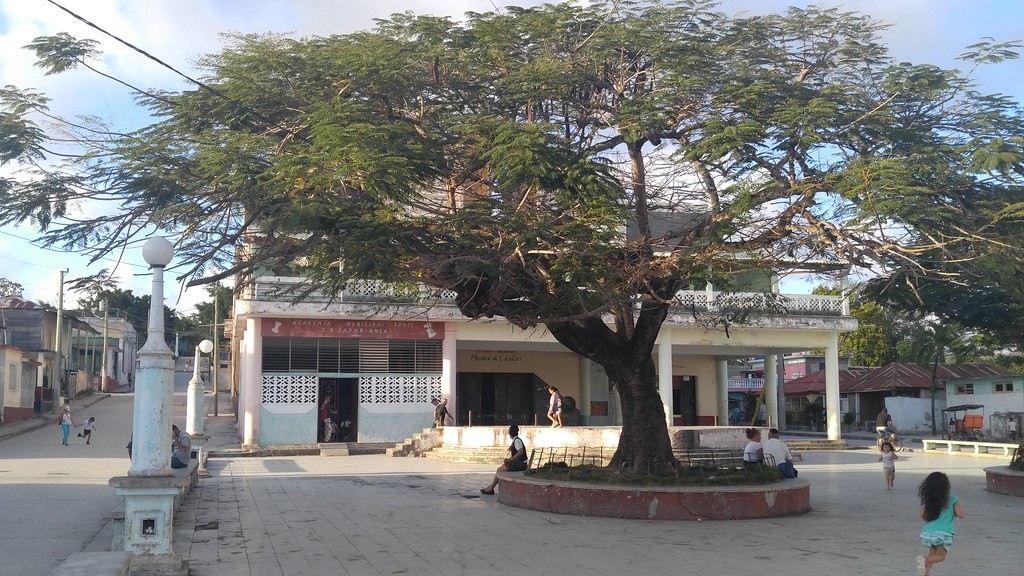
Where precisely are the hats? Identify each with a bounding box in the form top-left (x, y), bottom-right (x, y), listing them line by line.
top-left (430, 398), bottom-right (437, 403)
top-left (882, 408), bottom-right (887, 413)
top-left (64, 406), bottom-right (70, 411)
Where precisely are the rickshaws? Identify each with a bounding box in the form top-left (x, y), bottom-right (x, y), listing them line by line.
top-left (940, 401), bottom-right (983, 442)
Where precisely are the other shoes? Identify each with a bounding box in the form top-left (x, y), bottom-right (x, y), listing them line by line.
top-left (480, 489), bottom-right (494, 495)
top-left (78, 433), bottom-right (81, 437)
top-left (66, 443), bottom-right (68, 446)
top-left (917, 555), bottom-right (928, 576)
top-left (886, 485), bottom-right (890, 489)
top-left (63, 438), bottom-right (65, 444)
top-left (890, 481), bottom-right (894, 487)
top-left (554, 422), bottom-right (560, 428)
top-left (86, 442), bottom-right (90, 444)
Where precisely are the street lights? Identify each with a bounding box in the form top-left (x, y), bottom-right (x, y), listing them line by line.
top-left (186, 340), bottom-right (214, 436)
top-left (125, 235), bottom-right (177, 478)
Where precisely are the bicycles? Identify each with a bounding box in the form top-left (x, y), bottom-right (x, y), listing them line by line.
top-left (874, 427), bottom-right (903, 455)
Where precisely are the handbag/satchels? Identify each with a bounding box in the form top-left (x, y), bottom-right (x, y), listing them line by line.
top-left (432, 421), bottom-right (437, 427)
top-left (553, 404), bottom-right (558, 412)
top-left (58, 412), bottom-right (63, 425)
top-left (778, 463), bottom-right (796, 479)
top-left (737, 417), bottom-right (740, 422)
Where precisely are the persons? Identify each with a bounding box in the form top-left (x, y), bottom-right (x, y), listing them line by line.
top-left (172, 424), bottom-right (191, 469)
top-left (879, 442), bottom-right (898, 490)
top-left (430, 398), bottom-right (454, 428)
top-left (876, 409), bottom-right (897, 447)
top-left (743, 428), bottom-right (792, 469)
top-left (61, 406), bottom-right (96, 445)
top-left (126, 434), bottom-right (132, 460)
top-left (1008, 417), bottom-right (1018, 443)
top-left (320, 395), bottom-right (339, 443)
top-left (916, 472), bottom-right (964, 576)
top-left (548, 386), bottom-right (562, 429)
top-left (480, 425), bottom-right (528, 495)
